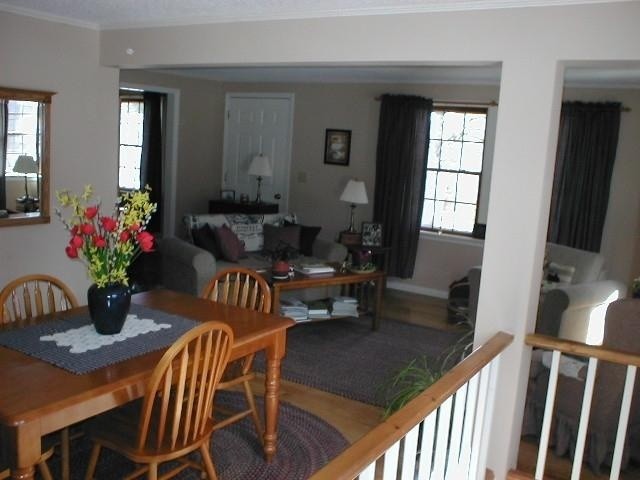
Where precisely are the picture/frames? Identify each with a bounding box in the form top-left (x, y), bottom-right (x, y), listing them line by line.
top-left (323, 126), bottom-right (353, 166)
top-left (219, 188), bottom-right (236, 202)
top-left (361, 220), bottom-right (385, 247)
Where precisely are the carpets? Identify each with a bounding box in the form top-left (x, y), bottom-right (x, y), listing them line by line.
top-left (239, 313), bottom-right (540, 438)
top-left (3, 383), bottom-right (351, 480)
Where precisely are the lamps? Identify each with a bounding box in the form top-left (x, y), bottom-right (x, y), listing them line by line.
top-left (248, 154), bottom-right (274, 205)
top-left (10, 154), bottom-right (38, 216)
top-left (338, 177), bottom-right (369, 236)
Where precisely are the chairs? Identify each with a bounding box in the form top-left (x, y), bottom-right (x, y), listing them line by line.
top-left (1, 271), bottom-right (105, 478)
top-left (525, 298), bottom-right (640, 475)
top-left (0, 442), bottom-right (56, 479)
top-left (78, 320), bottom-right (237, 480)
top-left (166, 271), bottom-right (276, 450)
top-left (464, 239), bottom-right (630, 349)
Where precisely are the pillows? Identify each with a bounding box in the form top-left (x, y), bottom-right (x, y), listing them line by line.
top-left (264, 221), bottom-right (301, 254)
top-left (192, 221), bottom-right (222, 259)
top-left (213, 224), bottom-right (247, 263)
top-left (547, 259), bottom-right (577, 285)
top-left (281, 217), bottom-right (321, 256)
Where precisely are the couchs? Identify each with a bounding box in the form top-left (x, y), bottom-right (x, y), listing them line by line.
top-left (160, 210), bottom-right (353, 310)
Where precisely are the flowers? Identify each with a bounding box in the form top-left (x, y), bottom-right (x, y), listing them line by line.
top-left (53, 178), bottom-right (161, 289)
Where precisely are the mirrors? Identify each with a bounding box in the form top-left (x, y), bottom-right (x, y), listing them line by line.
top-left (0, 86), bottom-right (59, 229)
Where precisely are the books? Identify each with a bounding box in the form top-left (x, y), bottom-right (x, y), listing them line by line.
top-left (279, 296), bottom-right (359, 322)
top-left (296, 261), bottom-right (335, 274)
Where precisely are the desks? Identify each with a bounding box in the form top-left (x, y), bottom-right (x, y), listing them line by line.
top-left (333, 238), bottom-right (394, 305)
top-left (206, 199), bottom-right (280, 216)
top-left (257, 262), bottom-right (391, 334)
top-left (1, 287), bottom-right (296, 478)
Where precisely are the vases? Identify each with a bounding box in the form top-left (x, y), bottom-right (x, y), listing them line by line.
top-left (88, 282), bottom-right (130, 336)
